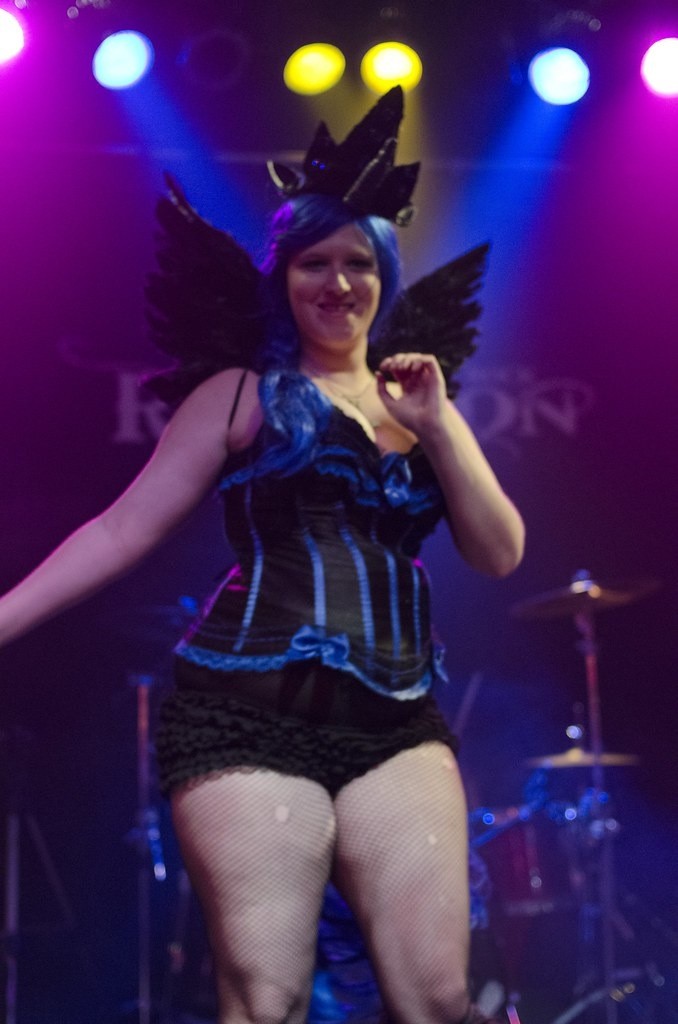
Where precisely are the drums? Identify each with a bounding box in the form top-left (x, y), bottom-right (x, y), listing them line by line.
top-left (468, 804), bottom-right (577, 917)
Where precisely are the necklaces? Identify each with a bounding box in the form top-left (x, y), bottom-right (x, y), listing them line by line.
top-left (304, 364), bottom-right (375, 403)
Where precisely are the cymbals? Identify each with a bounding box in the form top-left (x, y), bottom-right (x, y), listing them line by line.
top-left (511, 573), bottom-right (660, 616)
top-left (516, 747), bottom-right (641, 768)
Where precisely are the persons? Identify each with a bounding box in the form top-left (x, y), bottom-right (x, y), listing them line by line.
top-left (0, 195), bottom-right (526, 1024)
